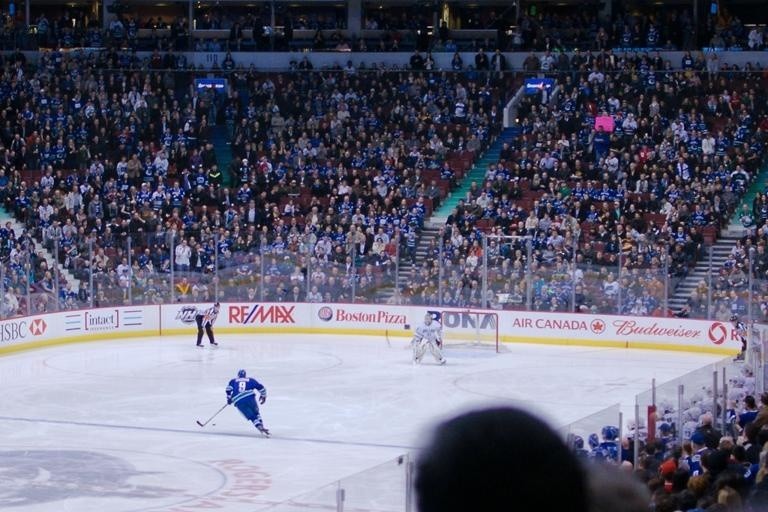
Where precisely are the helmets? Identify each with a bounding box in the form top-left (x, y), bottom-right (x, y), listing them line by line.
top-left (731, 365), bottom-right (755, 401)
top-left (214, 301), bottom-right (220, 311)
top-left (425, 313), bottom-right (431, 325)
top-left (626, 385), bottom-right (722, 439)
top-left (574, 426), bottom-right (619, 448)
top-left (238, 370), bottom-right (246, 377)
top-left (731, 315), bottom-right (737, 321)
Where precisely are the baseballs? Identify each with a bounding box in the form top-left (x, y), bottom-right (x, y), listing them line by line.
top-left (376, 261), bottom-right (379, 265)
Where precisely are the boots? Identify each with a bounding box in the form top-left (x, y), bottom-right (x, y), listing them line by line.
top-left (258, 420), bottom-right (268, 432)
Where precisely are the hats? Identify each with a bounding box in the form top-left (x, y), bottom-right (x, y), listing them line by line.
top-left (691, 433), bottom-right (705, 444)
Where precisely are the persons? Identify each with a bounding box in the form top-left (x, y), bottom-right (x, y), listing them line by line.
top-left (410, 314), bottom-right (446, 365)
top-left (225, 370), bottom-right (270, 435)
top-left (730, 315), bottom-right (762, 362)
top-left (564, 357), bottom-right (768, 512)
top-left (413, 406), bottom-right (591, 512)
top-left (0, 0), bottom-right (768, 322)
top-left (195, 302), bottom-right (222, 347)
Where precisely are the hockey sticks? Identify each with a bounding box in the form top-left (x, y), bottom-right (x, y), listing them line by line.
top-left (196, 402), bottom-right (229, 427)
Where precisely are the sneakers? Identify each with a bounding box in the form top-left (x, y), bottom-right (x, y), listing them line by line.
top-left (733, 354), bottom-right (744, 361)
top-left (197, 342), bottom-right (218, 347)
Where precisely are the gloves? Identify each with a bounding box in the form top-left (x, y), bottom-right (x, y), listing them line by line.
top-left (259, 396), bottom-right (265, 404)
top-left (228, 399), bottom-right (232, 404)
